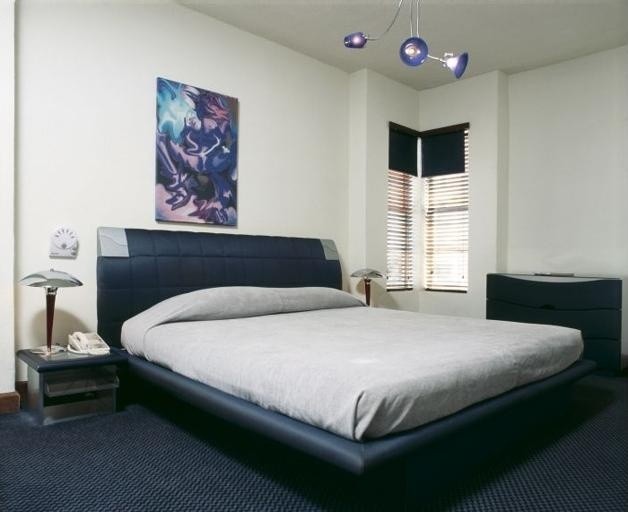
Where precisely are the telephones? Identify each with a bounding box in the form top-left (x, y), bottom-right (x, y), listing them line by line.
top-left (68, 331), bottom-right (111, 355)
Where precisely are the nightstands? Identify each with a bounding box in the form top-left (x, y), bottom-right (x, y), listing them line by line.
top-left (20, 350), bottom-right (126, 422)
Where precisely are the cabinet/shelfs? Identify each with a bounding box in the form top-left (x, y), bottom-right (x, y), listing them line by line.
top-left (486, 273), bottom-right (621, 376)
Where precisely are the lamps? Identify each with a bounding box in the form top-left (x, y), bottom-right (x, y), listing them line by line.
top-left (349, 268), bottom-right (383, 307)
top-left (341, 0), bottom-right (469, 79)
top-left (18, 267), bottom-right (83, 356)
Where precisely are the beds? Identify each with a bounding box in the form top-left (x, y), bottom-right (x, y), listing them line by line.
top-left (96, 228), bottom-right (598, 512)
